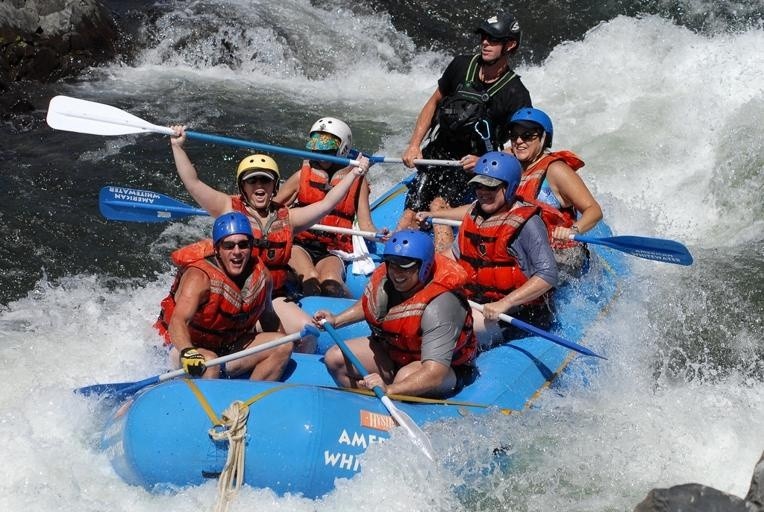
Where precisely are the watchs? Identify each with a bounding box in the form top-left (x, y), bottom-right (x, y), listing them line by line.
top-left (570, 225), bottom-right (581, 233)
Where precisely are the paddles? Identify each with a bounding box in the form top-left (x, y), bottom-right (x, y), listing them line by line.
top-left (345, 149), bottom-right (467, 173)
top-left (466, 296), bottom-right (605, 359)
top-left (74, 325), bottom-right (319, 404)
top-left (45, 96), bottom-right (364, 171)
top-left (416, 214), bottom-right (693, 267)
top-left (99, 185), bottom-right (390, 243)
top-left (317, 315), bottom-right (433, 464)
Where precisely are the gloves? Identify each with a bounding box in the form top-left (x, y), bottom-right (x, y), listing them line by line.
top-left (180, 347), bottom-right (206, 379)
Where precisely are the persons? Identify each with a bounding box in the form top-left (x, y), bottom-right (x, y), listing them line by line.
top-left (169, 125), bottom-right (370, 355)
top-left (151, 212), bottom-right (303, 382)
top-left (270, 117), bottom-right (394, 299)
top-left (441, 151), bottom-right (560, 350)
top-left (394, 10), bottom-right (534, 234)
top-left (415, 108), bottom-right (604, 288)
top-left (311, 229), bottom-right (479, 404)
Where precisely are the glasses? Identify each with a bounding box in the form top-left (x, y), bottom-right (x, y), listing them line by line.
top-left (220, 240), bottom-right (249, 250)
top-left (509, 131), bottom-right (545, 140)
top-left (246, 175), bottom-right (271, 185)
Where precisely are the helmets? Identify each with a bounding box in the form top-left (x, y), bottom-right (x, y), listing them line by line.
top-left (480, 11), bottom-right (520, 44)
top-left (212, 213), bottom-right (254, 246)
top-left (467, 152), bottom-right (522, 203)
top-left (382, 229), bottom-right (434, 283)
top-left (308, 117), bottom-right (352, 158)
top-left (506, 106), bottom-right (553, 146)
top-left (236, 154), bottom-right (280, 185)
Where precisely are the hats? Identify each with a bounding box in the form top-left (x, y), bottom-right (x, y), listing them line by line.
top-left (379, 255), bottom-right (419, 268)
top-left (305, 131), bottom-right (341, 151)
top-left (504, 120), bottom-right (540, 130)
top-left (467, 173), bottom-right (506, 187)
top-left (241, 169), bottom-right (276, 181)
top-left (474, 23), bottom-right (502, 39)
